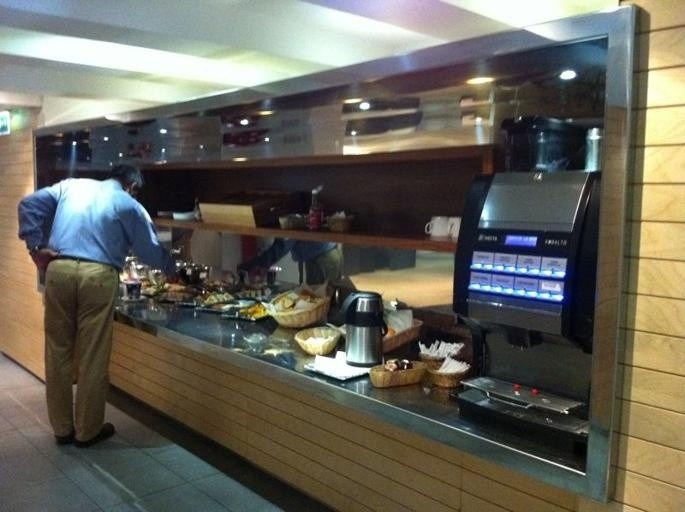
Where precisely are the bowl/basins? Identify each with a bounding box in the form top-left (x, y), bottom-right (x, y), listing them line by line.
top-left (157, 211), bottom-right (196, 222)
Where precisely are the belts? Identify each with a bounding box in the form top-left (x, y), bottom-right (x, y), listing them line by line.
top-left (52, 255), bottom-right (90, 261)
top-left (322, 245), bottom-right (338, 256)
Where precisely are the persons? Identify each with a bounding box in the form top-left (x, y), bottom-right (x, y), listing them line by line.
top-left (236, 237), bottom-right (359, 297)
top-left (16, 164), bottom-right (179, 449)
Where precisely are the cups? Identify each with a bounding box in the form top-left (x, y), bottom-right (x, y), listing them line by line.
top-left (425, 216), bottom-right (462, 237)
top-left (125, 281), bottom-right (142, 298)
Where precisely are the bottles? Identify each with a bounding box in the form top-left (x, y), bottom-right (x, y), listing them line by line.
top-left (308, 188), bottom-right (323, 230)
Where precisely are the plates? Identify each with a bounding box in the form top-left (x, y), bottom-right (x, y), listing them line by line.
top-left (120, 295), bottom-right (147, 302)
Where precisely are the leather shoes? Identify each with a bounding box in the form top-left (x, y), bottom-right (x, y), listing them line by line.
top-left (54, 423), bottom-right (115, 448)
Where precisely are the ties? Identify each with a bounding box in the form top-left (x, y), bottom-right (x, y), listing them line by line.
top-left (298, 258), bottom-right (303, 285)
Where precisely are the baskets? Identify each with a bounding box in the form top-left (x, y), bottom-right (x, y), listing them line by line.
top-left (325, 215), bottom-right (356, 233)
top-left (295, 327), bottom-right (341, 356)
top-left (268, 287), bottom-right (330, 329)
top-left (369, 319), bottom-right (471, 388)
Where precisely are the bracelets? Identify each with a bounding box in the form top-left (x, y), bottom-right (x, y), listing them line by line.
top-left (28, 244), bottom-right (47, 253)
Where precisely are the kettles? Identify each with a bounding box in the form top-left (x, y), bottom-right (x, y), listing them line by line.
top-left (337, 291), bottom-right (389, 366)
